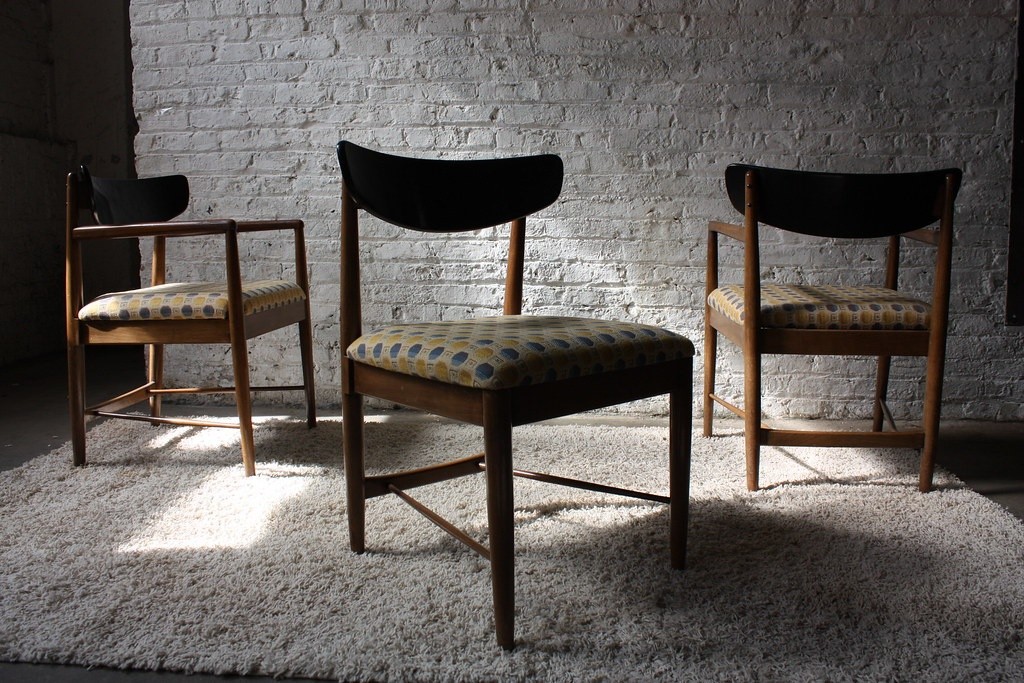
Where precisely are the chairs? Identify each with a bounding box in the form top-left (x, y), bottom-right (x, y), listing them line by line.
top-left (704, 161), bottom-right (965, 493)
top-left (336, 140), bottom-right (695, 651)
top-left (66, 162), bottom-right (317, 474)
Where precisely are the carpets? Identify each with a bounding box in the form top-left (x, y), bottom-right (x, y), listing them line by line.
top-left (0, 412), bottom-right (1024, 682)
